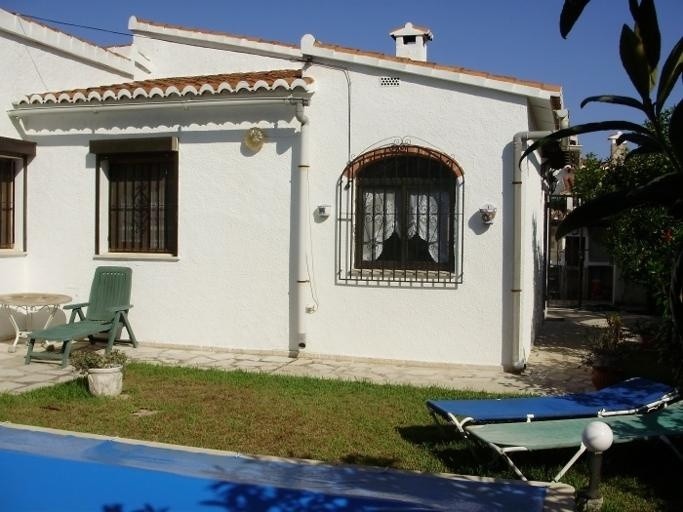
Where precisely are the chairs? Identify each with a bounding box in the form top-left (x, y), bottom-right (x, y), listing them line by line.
top-left (23, 265), bottom-right (137, 369)
top-left (424, 376), bottom-right (683, 482)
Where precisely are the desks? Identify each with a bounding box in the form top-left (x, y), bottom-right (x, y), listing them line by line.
top-left (0, 293), bottom-right (72, 352)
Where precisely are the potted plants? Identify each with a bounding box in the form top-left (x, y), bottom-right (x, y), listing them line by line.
top-left (69, 348), bottom-right (129, 398)
top-left (585, 325), bottom-right (625, 390)
